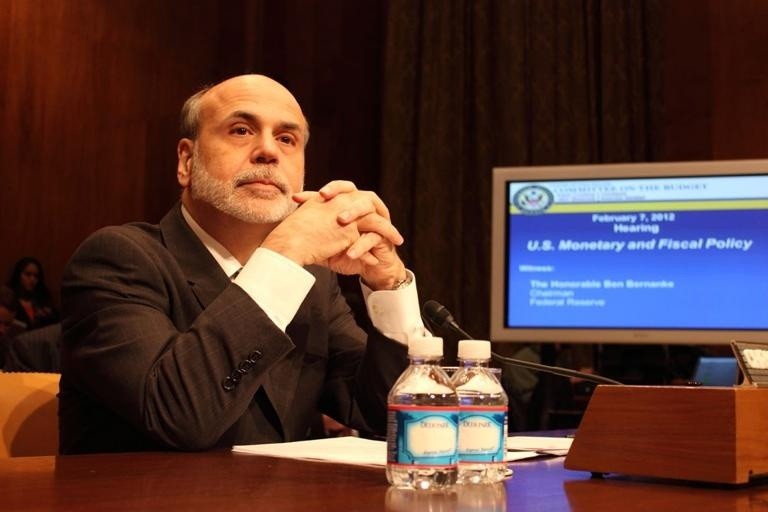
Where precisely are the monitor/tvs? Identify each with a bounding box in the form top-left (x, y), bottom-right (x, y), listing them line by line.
top-left (488, 157), bottom-right (768, 350)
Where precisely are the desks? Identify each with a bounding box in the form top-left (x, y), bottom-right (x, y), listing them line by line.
top-left (0, 429), bottom-right (768, 512)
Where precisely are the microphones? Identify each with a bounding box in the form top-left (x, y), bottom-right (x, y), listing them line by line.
top-left (421, 299), bottom-right (704, 386)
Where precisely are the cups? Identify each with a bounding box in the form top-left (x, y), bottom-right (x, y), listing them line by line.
top-left (435, 364), bottom-right (503, 383)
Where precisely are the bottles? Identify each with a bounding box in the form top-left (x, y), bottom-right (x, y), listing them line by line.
top-left (383, 336), bottom-right (459, 494)
top-left (449, 339), bottom-right (509, 486)
top-left (386, 481), bottom-right (506, 512)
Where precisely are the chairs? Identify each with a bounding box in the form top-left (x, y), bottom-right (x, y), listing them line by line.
top-left (0, 372), bottom-right (359, 460)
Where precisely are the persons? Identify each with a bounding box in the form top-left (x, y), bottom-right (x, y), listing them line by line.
top-left (57, 71), bottom-right (436, 453)
top-left (6, 256), bottom-right (60, 331)
top-left (499, 342), bottom-right (742, 433)
top-left (0, 300), bottom-right (62, 373)
